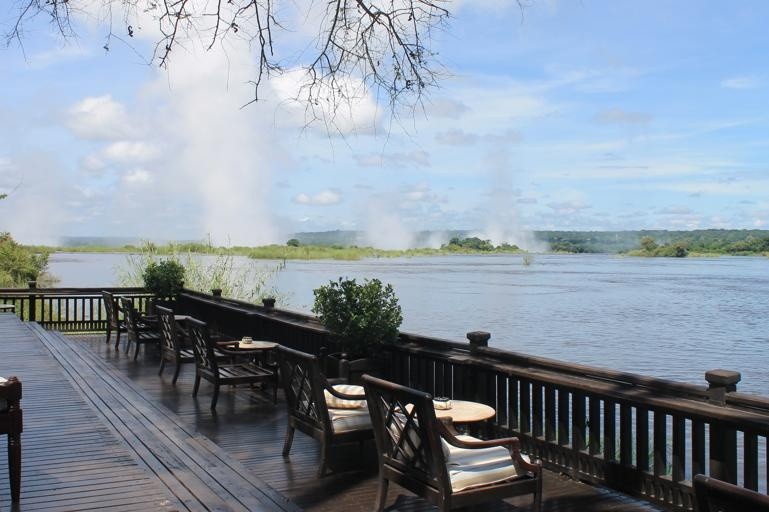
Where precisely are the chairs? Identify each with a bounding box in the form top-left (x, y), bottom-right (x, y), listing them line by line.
top-left (358, 370), bottom-right (543, 510)
top-left (185, 316), bottom-right (275, 413)
top-left (272, 340), bottom-right (379, 480)
top-left (119, 295), bottom-right (162, 361)
top-left (101, 289), bottom-right (146, 349)
top-left (155, 305), bottom-right (234, 387)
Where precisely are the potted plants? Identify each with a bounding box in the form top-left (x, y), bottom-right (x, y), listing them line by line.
top-left (310, 274), bottom-right (403, 383)
top-left (141, 257), bottom-right (189, 315)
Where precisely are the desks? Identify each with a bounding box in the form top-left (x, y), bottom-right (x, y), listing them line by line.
top-left (166, 312), bottom-right (191, 323)
top-left (393, 392), bottom-right (498, 428)
top-left (223, 339), bottom-right (276, 355)
